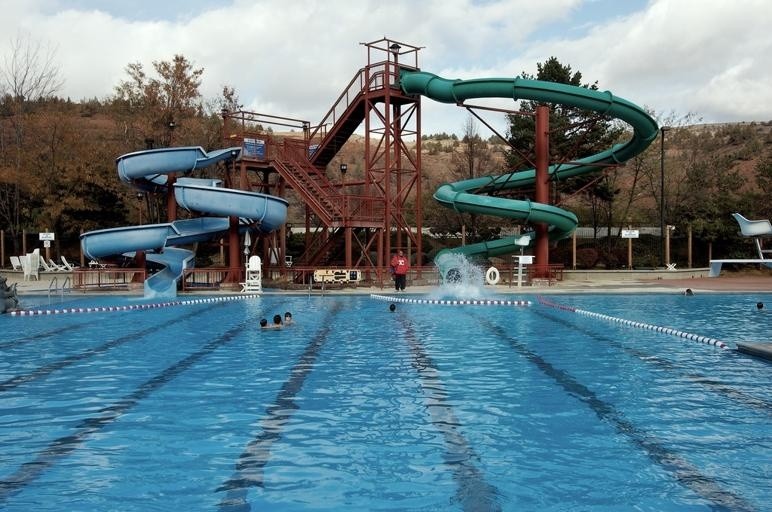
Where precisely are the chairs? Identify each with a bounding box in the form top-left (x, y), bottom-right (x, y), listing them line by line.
top-left (9, 249), bottom-right (80, 281)
top-left (240, 256), bottom-right (262, 293)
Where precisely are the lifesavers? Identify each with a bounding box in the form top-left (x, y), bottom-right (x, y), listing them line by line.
top-left (485, 266), bottom-right (500, 285)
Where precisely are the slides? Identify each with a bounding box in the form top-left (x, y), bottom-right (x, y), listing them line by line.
top-left (80, 146), bottom-right (289, 299)
top-left (399, 71), bottom-right (659, 286)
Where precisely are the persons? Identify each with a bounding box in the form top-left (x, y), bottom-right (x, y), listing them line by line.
top-left (271, 315), bottom-right (285, 327)
top-left (282, 311), bottom-right (296, 325)
top-left (757, 302), bottom-right (763, 309)
top-left (390, 249), bottom-right (409, 292)
top-left (260, 319), bottom-right (272, 328)
top-left (386, 304), bottom-right (397, 313)
top-left (682, 287), bottom-right (695, 297)
